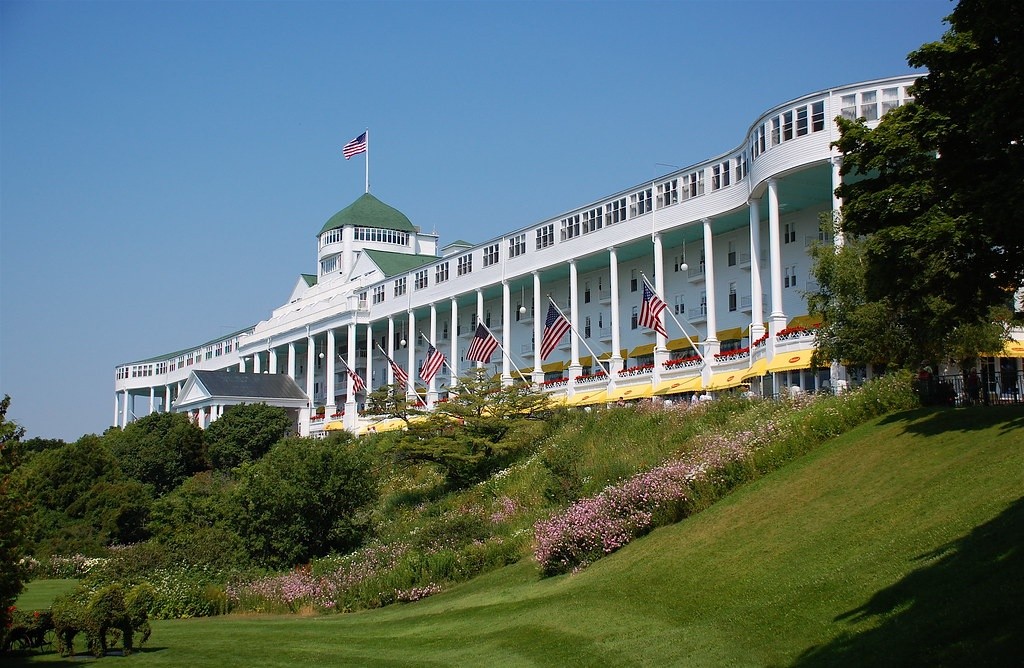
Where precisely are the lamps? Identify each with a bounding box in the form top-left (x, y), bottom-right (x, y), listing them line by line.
top-left (263, 359), bottom-right (268, 374)
top-left (400, 322), bottom-right (406, 345)
top-left (519, 285), bottom-right (526, 314)
top-left (680, 232), bottom-right (689, 270)
top-left (171, 387), bottom-right (176, 404)
top-left (319, 340), bottom-right (324, 358)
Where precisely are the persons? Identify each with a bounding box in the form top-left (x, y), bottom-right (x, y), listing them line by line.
top-left (966, 367), bottom-right (989, 406)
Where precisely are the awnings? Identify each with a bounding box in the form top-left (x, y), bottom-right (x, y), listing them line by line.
top-left (444, 312), bottom-right (835, 390)
top-left (316, 407), bottom-right (324, 413)
top-left (416, 388), bottom-right (426, 396)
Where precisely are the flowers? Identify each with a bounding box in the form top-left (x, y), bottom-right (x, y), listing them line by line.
top-left (540, 316), bottom-right (822, 385)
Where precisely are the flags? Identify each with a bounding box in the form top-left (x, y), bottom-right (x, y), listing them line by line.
top-left (540, 301), bottom-right (571, 361)
top-left (378, 349), bottom-right (408, 390)
top-left (465, 323), bottom-right (499, 364)
top-left (341, 130), bottom-right (366, 160)
top-left (638, 278), bottom-right (669, 340)
top-left (419, 343), bottom-right (444, 385)
top-left (340, 360), bottom-right (363, 395)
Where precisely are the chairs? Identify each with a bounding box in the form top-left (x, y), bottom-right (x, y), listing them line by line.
top-left (981, 383), bottom-right (998, 403)
